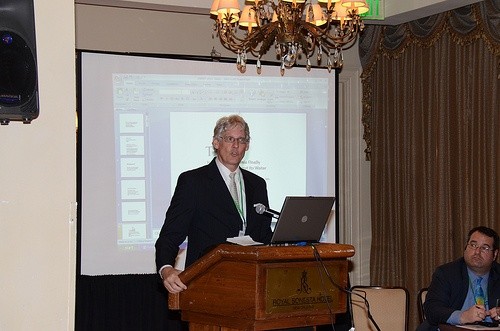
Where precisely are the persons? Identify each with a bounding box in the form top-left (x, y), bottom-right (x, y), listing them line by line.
top-left (155, 115), bottom-right (272, 295)
top-left (416, 226), bottom-right (500, 331)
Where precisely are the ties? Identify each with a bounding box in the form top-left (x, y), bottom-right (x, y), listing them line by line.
top-left (475, 277), bottom-right (485, 305)
top-left (229, 173), bottom-right (239, 206)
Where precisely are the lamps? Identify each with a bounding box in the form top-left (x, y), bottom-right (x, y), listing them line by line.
top-left (208, 0), bottom-right (370, 77)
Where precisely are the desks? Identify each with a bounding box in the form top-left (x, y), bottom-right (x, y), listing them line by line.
top-left (437, 321), bottom-right (500, 331)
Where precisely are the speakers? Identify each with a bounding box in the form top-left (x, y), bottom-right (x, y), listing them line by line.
top-left (0, 0), bottom-right (40, 125)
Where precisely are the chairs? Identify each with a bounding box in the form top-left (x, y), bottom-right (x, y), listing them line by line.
top-left (417, 287), bottom-right (441, 331)
top-left (347, 285), bottom-right (410, 331)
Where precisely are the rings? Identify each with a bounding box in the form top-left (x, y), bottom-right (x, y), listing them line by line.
top-left (478, 312), bottom-right (480, 316)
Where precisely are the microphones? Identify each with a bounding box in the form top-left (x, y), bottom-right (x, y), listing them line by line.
top-left (255, 203), bottom-right (280, 219)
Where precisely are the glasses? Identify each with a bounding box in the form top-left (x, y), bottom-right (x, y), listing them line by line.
top-left (220, 135), bottom-right (247, 145)
top-left (465, 241), bottom-right (493, 253)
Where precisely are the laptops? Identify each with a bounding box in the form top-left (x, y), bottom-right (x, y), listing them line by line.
top-left (263, 195), bottom-right (335, 245)
